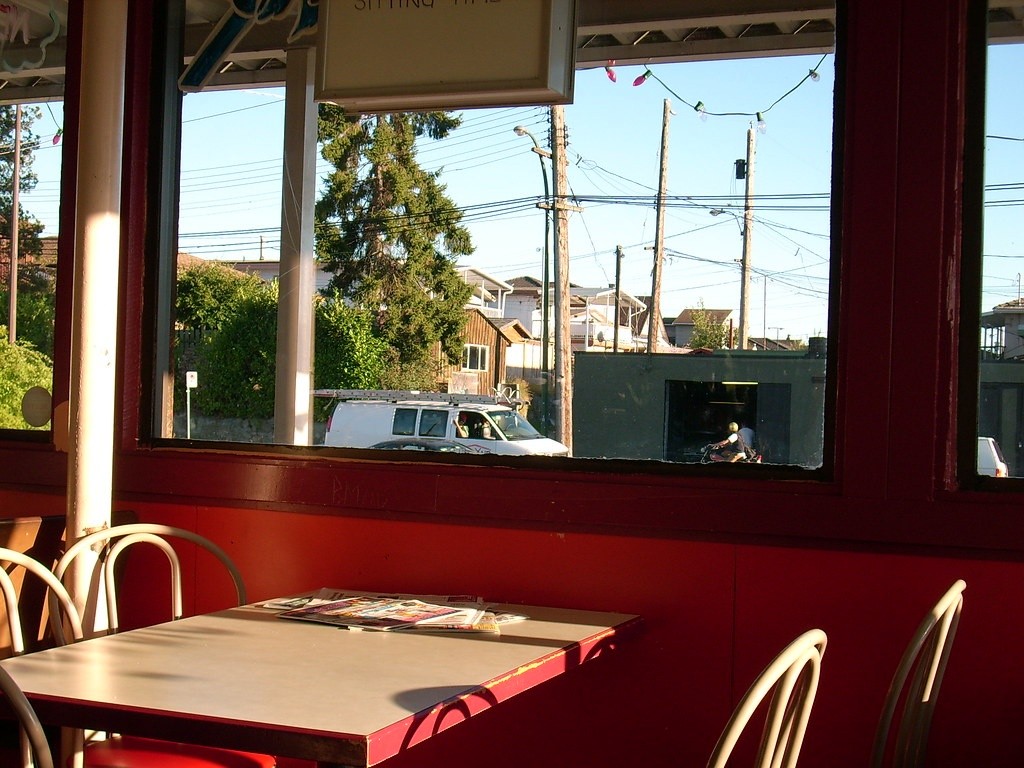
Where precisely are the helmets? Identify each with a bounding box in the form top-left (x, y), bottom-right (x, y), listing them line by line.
top-left (729, 422), bottom-right (739, 432)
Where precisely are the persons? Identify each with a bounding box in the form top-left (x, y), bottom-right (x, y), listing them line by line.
top-left (714, 422), bottom-right (746, 462)
top-left (453, 412), bottom-right (469, 437)
top-left (479, 416), bottom-right (496, 439)
top-left (739, 420), bottom-right (755, 447)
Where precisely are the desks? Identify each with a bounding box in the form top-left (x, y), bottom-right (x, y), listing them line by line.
top-left (6, 586), bottom-right (642, 768)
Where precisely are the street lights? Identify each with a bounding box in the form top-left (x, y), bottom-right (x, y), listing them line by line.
top-left (513, 126), bottom-right (550, 436)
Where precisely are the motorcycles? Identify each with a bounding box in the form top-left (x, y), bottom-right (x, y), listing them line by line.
top-left (697, 442), bottom-right (762, 463)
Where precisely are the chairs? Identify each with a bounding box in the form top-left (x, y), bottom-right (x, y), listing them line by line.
top-left (0, 524), bottom-right (321, 768)
top-left (706, 578), bottom-right (969, 768)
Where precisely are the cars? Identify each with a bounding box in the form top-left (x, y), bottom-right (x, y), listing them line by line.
top-left (369, 436), bottom-right (473, 454)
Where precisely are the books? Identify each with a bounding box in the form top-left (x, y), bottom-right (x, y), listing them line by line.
top-left (263, 586), bottom-right (531, 634)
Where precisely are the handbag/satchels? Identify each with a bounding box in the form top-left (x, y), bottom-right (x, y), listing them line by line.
top-left (743, 445), bottom-right (755, 460)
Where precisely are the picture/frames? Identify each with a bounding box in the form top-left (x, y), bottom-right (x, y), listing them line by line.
top-left (312, 0), bottom-right (579, 117)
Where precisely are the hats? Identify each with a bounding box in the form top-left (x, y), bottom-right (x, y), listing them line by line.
top-left (460, 413), bottom-right (466, 422)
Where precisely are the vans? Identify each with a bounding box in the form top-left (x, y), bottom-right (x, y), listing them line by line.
top-left (325, 400), bottom-right (569, 457)
top-left (976, 437), bottom-right (1008, 478)
